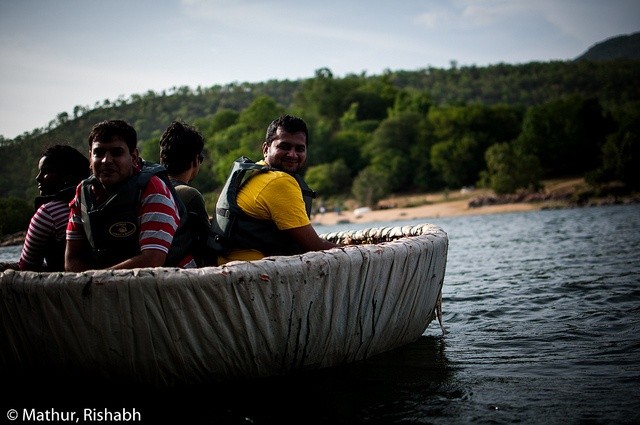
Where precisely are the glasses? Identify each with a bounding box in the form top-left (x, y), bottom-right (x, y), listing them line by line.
top-left (197, 154), bottom-right (203, 163)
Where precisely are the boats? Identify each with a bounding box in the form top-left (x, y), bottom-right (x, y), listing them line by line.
top-left (0, 223), bottom-right (449, 387)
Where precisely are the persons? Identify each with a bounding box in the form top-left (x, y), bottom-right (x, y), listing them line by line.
top-left (0, 145), bottom-right (90, 271)
top-left (208, 116), bottom-right (341, 266)
top-left (64, 120), bottom-right (188, 272)
top-left (158, 121), bottom-right (218, 269)
